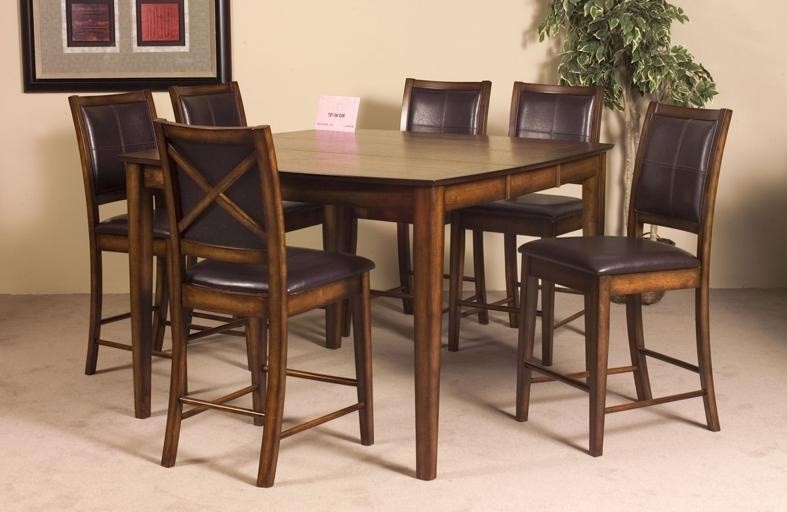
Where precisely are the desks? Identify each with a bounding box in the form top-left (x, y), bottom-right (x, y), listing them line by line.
top-left (116, 128), bottom-right (615, 480)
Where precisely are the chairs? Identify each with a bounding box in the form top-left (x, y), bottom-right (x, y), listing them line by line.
top-left (516, 100), bottom-right (733, 457)
top-left (68, 89), bottom-right (251, 375)
top-left (153, 117), bottom-right (375, 488)
top-left (447, 81), bottom-right (603, 366)
top-left (151, 81), bottom-right (325, 351)
top-left (339, 79), bottom-right (493, 352)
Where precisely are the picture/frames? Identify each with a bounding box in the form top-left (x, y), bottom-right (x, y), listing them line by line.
top-left (19, 0), bottom-right (232, 93)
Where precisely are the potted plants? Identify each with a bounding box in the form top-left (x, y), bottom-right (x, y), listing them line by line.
top-left (537, 0), bottom-right (720, 305)
